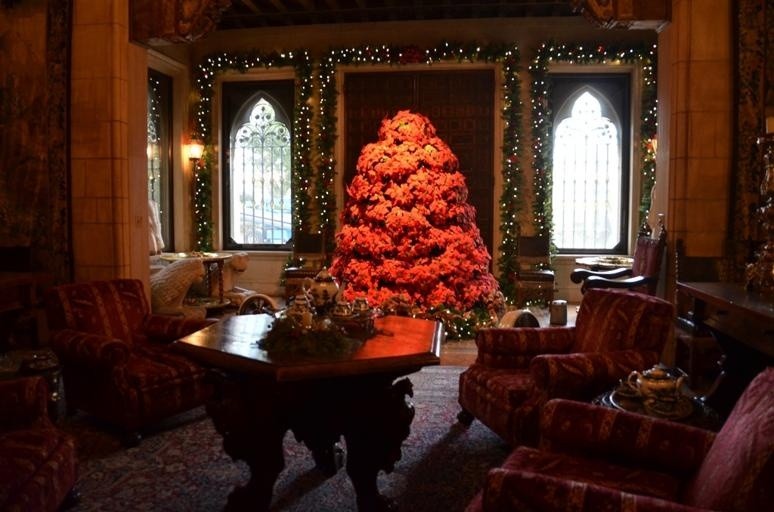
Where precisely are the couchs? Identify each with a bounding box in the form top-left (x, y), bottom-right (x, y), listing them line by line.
top-left (457, 280), bottom-right (675, 450)
top-left (1, 376), bottom-right (83, 509)
top-left (471, 369), bottom-right (774, 510)
top-left (35, 279), bottom-right (213, 446)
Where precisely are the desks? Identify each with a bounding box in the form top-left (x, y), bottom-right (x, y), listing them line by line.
top-left (4, 347), bottom-right (65, 420)
top-left (597, 384), bottom-right (723, 435)
top-left (159, 252), bottom-right (234, 311)
top-left (175, 315), bottom-right (446, 506)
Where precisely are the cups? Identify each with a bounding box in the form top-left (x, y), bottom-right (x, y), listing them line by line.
top-left (334, 296), bottom-right (370, 317)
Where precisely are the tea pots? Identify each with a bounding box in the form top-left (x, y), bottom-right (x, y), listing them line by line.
top-left (303, 266), bottom-right (347, 315)
top-left (628, 364), bottom-right (688, 403)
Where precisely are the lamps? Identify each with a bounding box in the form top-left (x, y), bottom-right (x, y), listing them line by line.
top-left (186, 134), bottom-right (204, 169)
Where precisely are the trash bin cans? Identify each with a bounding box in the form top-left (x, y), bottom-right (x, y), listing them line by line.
top-left (551, 300), bottom-right (567, 325)
